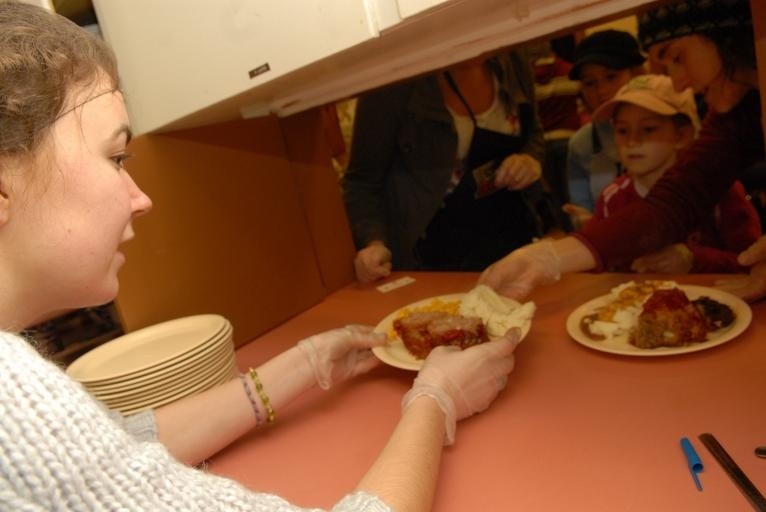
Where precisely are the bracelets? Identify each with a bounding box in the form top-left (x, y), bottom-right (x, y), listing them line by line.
top-left (238, 373), bottom-right (264, 430)
top-left (249, 368), bottom-right (276, 425)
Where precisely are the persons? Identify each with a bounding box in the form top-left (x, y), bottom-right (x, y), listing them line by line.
top-left (596, 75), bottom-right (760, 274)
top-left (341, 48), bottom-right (563, 283)
top-left (567, 31), bottom-right (648, 213)
top-left (475, 1), bottom-right (766, 310)
top-left (0, 0), bottom-right (521, 512)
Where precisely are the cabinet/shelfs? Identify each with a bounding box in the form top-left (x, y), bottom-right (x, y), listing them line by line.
top-left (87, 1), bottom-right (630, 139)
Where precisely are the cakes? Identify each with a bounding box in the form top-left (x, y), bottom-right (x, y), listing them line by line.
top-left (392, 309), bottom-right (491, 360)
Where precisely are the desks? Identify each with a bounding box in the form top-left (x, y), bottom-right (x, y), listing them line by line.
top-left (198, 268), bottom-right (766, 512)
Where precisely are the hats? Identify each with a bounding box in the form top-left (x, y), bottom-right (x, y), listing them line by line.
top-left (590, 73), bottom-right (702, 142)
top-left (567, 29), bottom-right (647, 81)
top-left (635, 1), bottom-right (753, 50)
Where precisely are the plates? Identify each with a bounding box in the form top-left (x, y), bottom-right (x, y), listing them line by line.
top-left (62, 313), bottom-right (241, 419)
top-left (370, 291), bottom-right (532, 374)
top-left (566, 285), bottom-right (753, 358)
